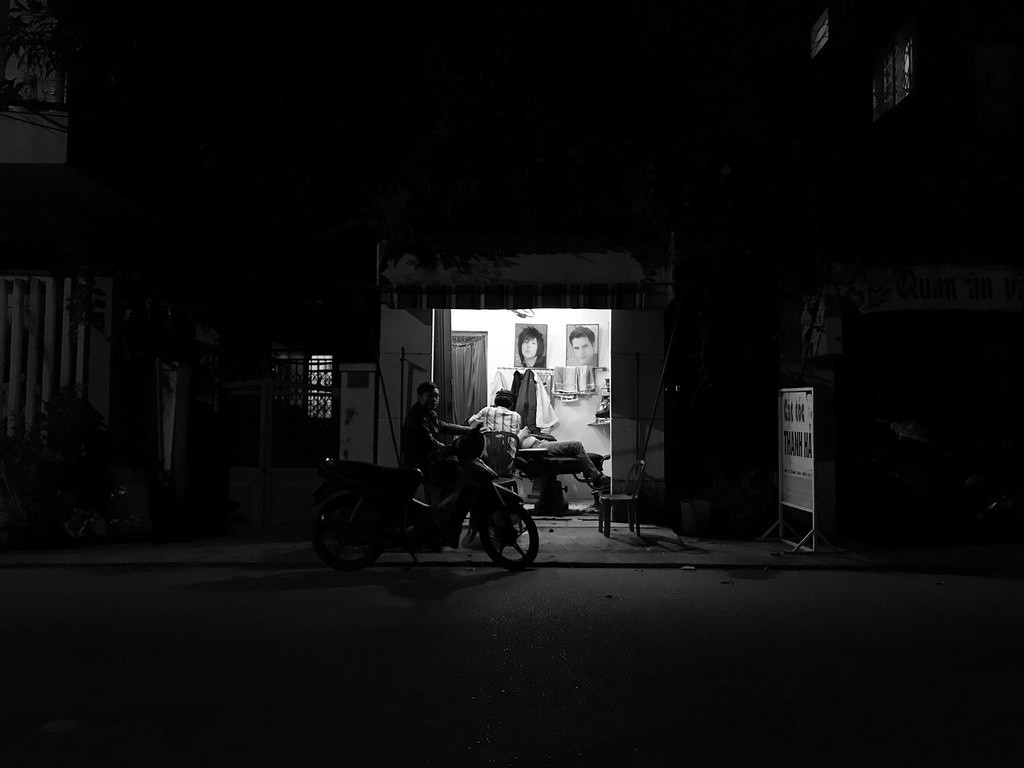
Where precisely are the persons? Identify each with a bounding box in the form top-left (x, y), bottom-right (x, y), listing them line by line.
top-left (468, 389), bottom-right (521, 472)
top-left (567, 326), bottom-right (598, 367)
top-left (402, 381), bottom-right (471, 504)
top-left (516, 426), bottom-right (610, 487)
top-left (518, 326), bottom-right (546, 367)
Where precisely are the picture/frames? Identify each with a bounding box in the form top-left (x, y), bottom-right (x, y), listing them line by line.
top-left (565, 323), bottom-right (599, 367)
top-left (514, 323), bottom-right (548, 369)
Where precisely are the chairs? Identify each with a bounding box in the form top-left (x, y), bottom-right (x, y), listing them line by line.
top-left (465, 431), bottom-right (520, 545)
top-left (515, 434), bottom-right (603, 516)
top-left (598, 459), bottom-right (645, 539)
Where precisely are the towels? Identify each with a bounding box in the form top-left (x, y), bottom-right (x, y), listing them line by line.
top-left (552, 365), bottom-right (597, 402)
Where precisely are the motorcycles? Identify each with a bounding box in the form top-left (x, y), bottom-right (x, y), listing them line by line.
top-left (301, 423), bottom-right (540, 572)
